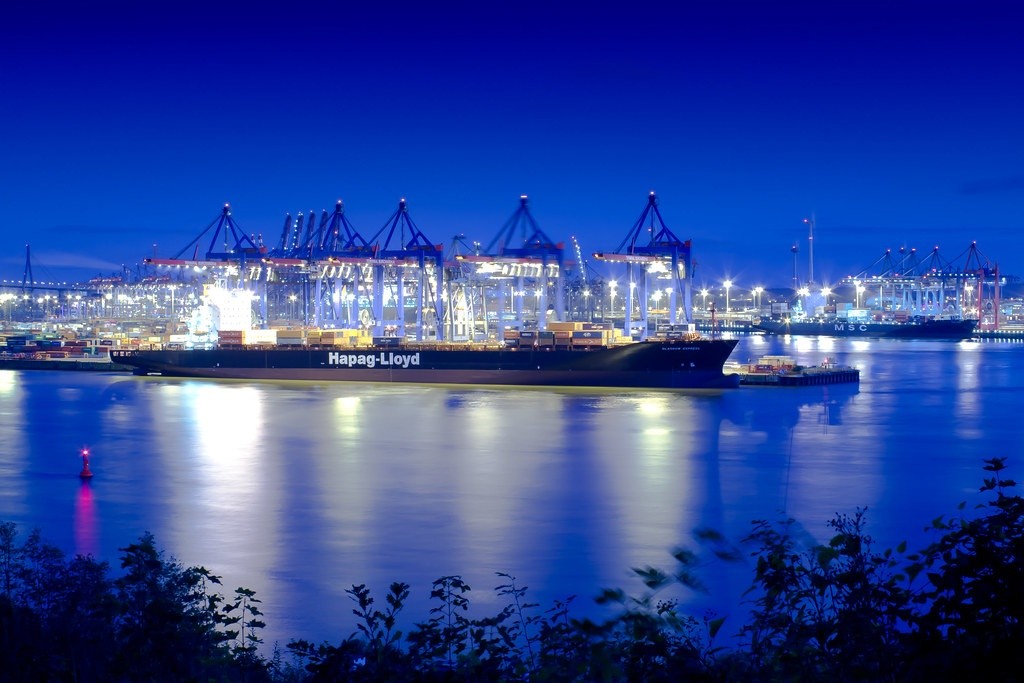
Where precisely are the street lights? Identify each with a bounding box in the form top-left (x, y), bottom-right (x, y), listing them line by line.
top-left (583, 280), bottom-right (764, 316)
top-left (853, 280), bottom-right (861, 309)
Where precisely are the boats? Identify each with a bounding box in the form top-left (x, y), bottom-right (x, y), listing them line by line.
top-left (752, 318), bottom-right (979, 339)
top-left (109, 338), bottom-right (739, 389)
